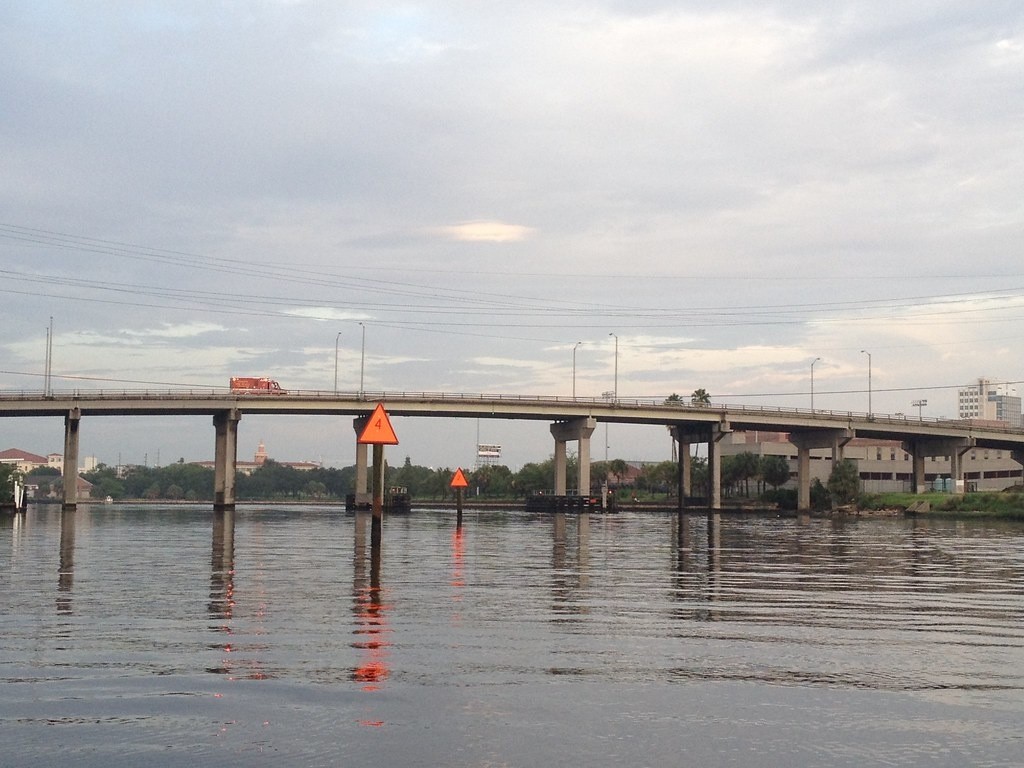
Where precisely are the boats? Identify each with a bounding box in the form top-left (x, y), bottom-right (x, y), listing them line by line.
top-left (345, 484), bottom-right (413, 516)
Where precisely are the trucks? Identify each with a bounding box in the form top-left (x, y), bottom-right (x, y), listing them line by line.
top-left (229, 376), bottom-right (289, 397)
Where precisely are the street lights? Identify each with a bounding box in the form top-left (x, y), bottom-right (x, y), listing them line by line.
top-left (861, 350), bottom-right (872, 419)
top-left (609, 332), bottom-right (618, 404)
top-left (48, 316), bottom-right (54, 394)
top-left (44, 327), bottom-right (49, 395)
top-left (334, 331), bottom-right (342, 394)
top-left (811, 357), bottom-right (821, 419)
top-left (572, 341), bottom-right (581, 400)
top-left (359, 322), bottom-right (366, 393)
